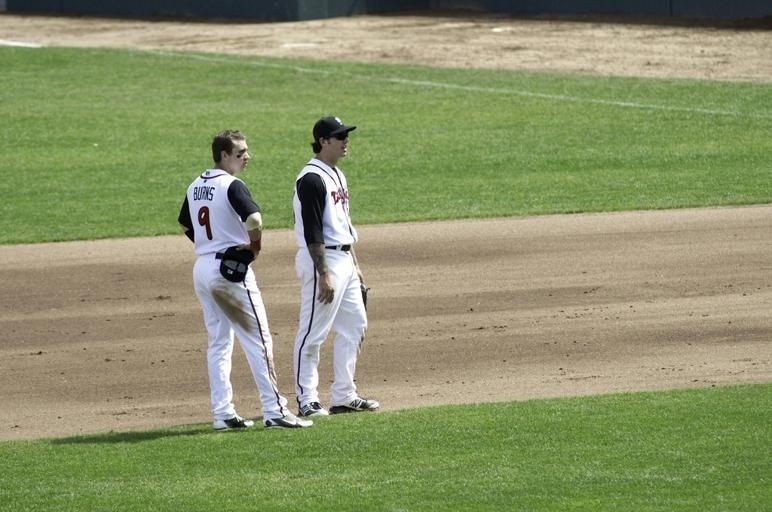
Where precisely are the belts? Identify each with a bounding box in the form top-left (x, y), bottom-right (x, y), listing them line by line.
top-left (325, 244), bottom-right (351, 251)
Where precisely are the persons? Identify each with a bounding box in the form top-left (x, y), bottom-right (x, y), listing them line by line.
top-left (178, 129), bottom-right (315, 431)
top-left (292, 115), bottom-right (381, 418)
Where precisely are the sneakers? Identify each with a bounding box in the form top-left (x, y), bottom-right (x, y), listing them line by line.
top-left (262, 401), bottom-right (329, 428)
top-left (329, 396), bottom-right (380, 414)
top-left (213, 415), bottom-right (254, 431)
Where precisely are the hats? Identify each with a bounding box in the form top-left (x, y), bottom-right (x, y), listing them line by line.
top-left (220, 244), bottom-right (255, 283)
top-left (312, 116), bottom-right (356, 141)
